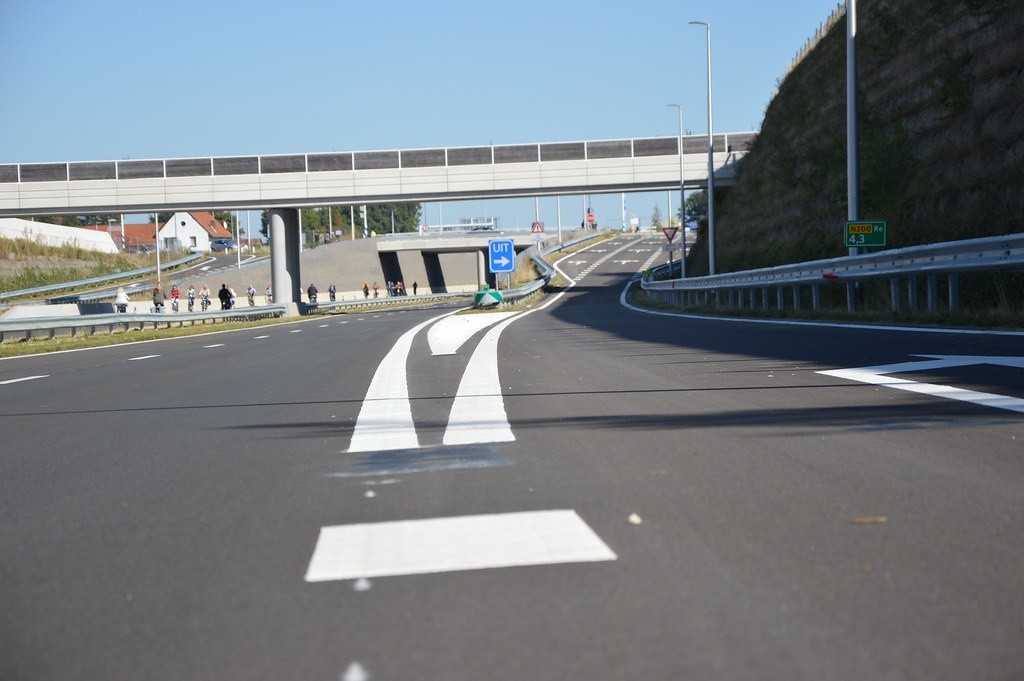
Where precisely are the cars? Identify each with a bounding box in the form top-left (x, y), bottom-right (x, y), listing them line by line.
top-left (210, 239), bottom-right (236, 252)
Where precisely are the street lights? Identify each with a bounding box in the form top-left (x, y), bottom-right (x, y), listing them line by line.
top-left (688, 20), bottom-right (715, 274)
top-left (667, 102), bottom-right (686, 279)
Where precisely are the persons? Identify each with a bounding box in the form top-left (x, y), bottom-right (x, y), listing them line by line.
top-left (186, 285), bottom-right (196, 310)
top-left (413, 281), bottom-right (417, 295)
top-left (307, 283), bottom-right (318, 302)
top-left (218, 283), bottom-right (237, 309)
top-left (113, 287), bottom-right (129, 313)
top-left (362, 281), bottom-right (370, 296)
top-left (153, 282), bottom-right (166, 310)
top-left (264, 286), bottom-right (273, 299)
top-left (387, 280), bottom-right (406, 296)
top-left (170, 285), bottom-right (181, 310)
top-left (373, 282), bottom-right (379, 296)
top-left (199, 284), bottom-right (211, 308)
top-left (245, 285), bottom-right (256, 304)
top-left (300, 284), bottom-right (304, 294)
top-left (328, 283), bottom-right (336, 301)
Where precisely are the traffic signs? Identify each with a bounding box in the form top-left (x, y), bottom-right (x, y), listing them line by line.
top-left (488, 238), bottom-right (514, 273)
